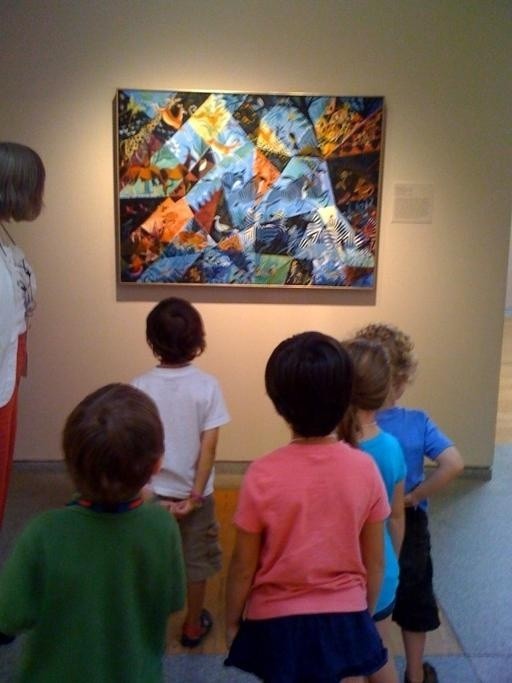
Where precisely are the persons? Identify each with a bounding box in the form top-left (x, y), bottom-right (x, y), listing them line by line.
top-left (0, 141), bottom-right (44, 515)
top-left (220, 331), bottom-right (392, 683)
top-left (357, 324), bottom-right (462, 682)
top-left (119, 298), bottom-right (229, 646)
top-left (342, 339), bottom-right (408, 682)
top-left (1, 386), bottom-right (189, 681)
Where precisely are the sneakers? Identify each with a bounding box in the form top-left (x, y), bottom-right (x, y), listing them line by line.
top-left (404, 662), bottom-right (438, 683)
top-left (181, 608), bottom-right (213, 648)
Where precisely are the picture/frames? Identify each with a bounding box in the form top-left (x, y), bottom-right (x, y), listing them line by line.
top-left (115, 86), bottom-right (388, 292)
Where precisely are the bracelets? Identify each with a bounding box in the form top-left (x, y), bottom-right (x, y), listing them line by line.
top-left (188, 491), bottom-right (204, 508)
